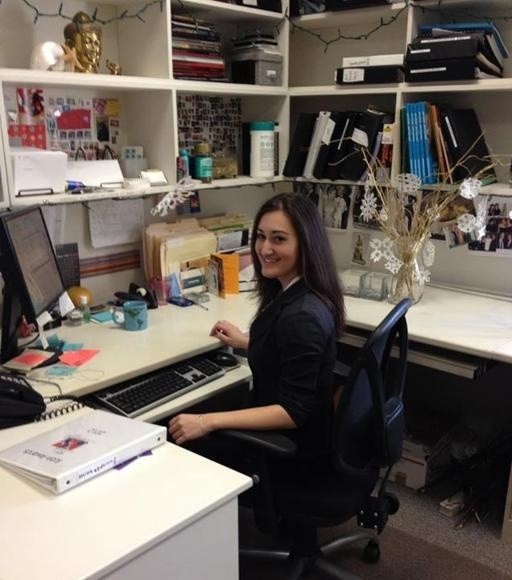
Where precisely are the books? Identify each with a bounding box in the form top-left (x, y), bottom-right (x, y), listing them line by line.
top-left (1, 406), bottom-right (168, 496)
top-left (171, 13), bottom-right (229, 82)
top-left (399, 101), bottom-right (496, 187)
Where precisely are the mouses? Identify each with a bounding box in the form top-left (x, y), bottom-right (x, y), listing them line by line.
top-left (208, 352), bottom-right (237, 366)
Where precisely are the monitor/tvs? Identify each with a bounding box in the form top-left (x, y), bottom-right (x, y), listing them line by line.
top-left (0, 203), bottom-right (65, 371)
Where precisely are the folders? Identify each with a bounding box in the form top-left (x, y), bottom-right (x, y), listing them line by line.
top-left (404, 17), bottom-right (509, 79)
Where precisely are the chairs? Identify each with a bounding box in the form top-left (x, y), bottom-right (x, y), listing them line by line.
top-left (219, 299), bottom-right (416, 579)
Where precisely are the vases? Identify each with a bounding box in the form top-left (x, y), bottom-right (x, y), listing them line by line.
top-left (387, 256), bottom-right (424, 308)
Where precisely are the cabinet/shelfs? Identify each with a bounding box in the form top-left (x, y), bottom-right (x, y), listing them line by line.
top-left (2, 0), bottom-right (511, 212)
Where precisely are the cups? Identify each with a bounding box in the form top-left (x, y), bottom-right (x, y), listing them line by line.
top-left (112, 300), bottom-right (148, 331)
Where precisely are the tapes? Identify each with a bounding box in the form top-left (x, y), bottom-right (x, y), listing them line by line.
top-left (135, 287), bottom-right (147, 297)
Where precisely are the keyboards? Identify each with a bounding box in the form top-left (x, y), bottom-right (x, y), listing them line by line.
top-left (92, 356), bottom-right (225, 418)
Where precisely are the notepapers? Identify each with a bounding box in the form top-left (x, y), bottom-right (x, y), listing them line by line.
top-left (90, 311), bottom-right (119, 323)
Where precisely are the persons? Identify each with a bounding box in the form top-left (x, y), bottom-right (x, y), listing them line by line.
top-left (63, 11), bottom-right (103, 74)
top-left (98, 122), bottom-right (109, 140)
top-left (404, 191), bottom-right (417, 231)
top-left (294, 183), bottom-right (351, 229)
top-left (451, 203), bottom-right (512, 251)
top-left (168, 191), bottom-right (346, 491)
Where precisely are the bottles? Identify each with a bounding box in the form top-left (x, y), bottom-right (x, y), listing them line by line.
top-left (249, 120), bottom-right (275, 178)
top-left (79, 296), bottom-right (91, 324)
top-left (179, 148), bottom-right (213, 184)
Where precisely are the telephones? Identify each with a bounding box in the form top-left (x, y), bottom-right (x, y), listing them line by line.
top-left (0, 374), bottom-right (47, 417)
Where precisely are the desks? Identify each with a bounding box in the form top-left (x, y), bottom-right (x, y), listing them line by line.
top-left (0, 269), bottom-right (512, 580)
